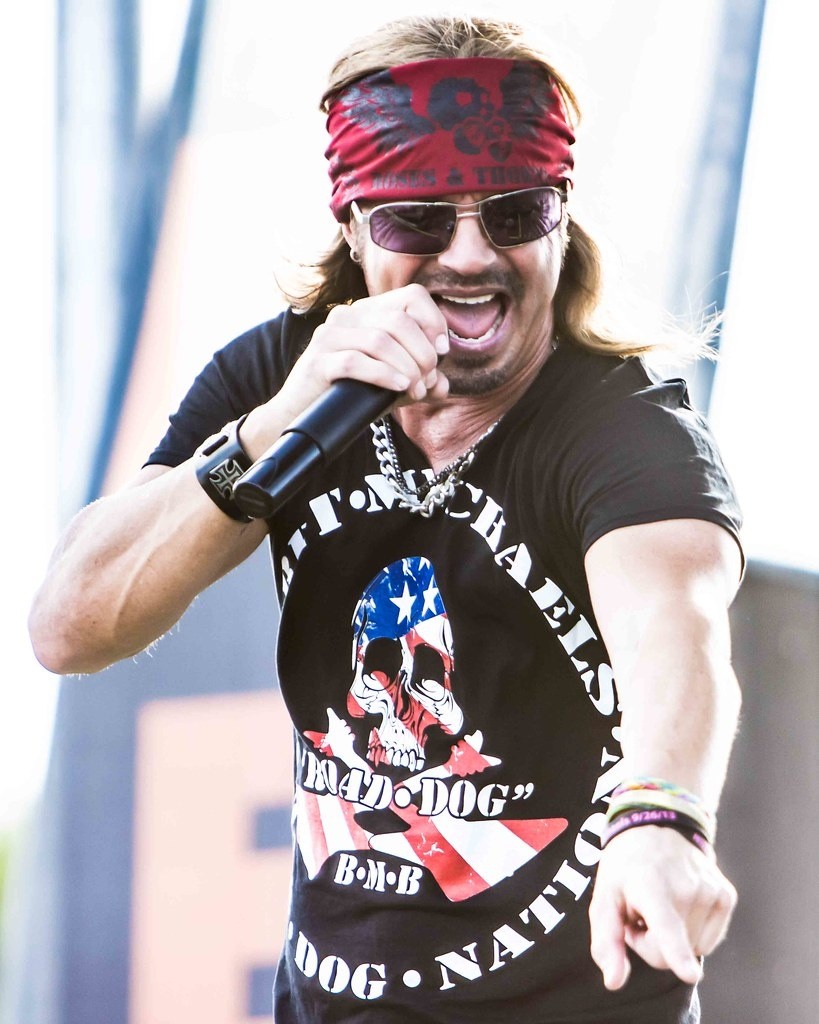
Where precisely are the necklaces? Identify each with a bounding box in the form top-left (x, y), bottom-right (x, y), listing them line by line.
top-left (367, 419), bottom-right (505, 495)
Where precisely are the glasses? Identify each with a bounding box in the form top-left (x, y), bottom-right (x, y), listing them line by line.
top-left (349, 182), bottom-right (569, 255)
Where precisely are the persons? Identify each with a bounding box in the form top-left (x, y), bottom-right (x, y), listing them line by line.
top-left (28, 14), bottom-right (749, 1024)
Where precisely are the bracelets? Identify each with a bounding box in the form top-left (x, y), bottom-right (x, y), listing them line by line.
top-left (190, 417), bottom-right (269, 527)
top-left (598, 780), bottom-right (716, 851)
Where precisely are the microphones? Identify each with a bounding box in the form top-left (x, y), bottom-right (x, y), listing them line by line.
top-left (230, 353), bottom-right (446, 518)
